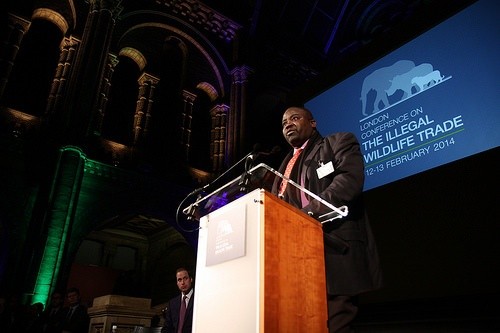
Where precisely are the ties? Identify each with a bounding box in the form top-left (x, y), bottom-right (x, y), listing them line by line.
top-left (178, 296), bottom-right (187, 332)
top-left (51, 310), bottom-right (56, 317)
top-left (280, 148), bottom-right (304, 195)
top-left (66, 308), bottom-right (73, 321)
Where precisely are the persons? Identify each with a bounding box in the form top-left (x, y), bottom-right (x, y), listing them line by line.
top-left (0, 288), bottom-right (90, 333)
top-left (161, 268), bottom-right (194, 333)
top-left (271, 107), bottom-right (381, 333)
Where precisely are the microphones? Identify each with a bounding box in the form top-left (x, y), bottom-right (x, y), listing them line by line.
top-left (245, 151), bottom-right (283, 172)
top-left (193, 142), bottom-right (260, 195)
